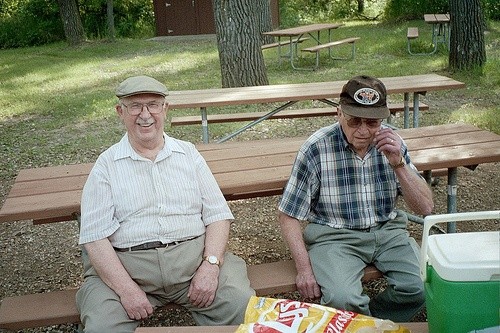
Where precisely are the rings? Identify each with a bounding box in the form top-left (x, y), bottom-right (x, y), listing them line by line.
top-left (390, 140), bottom-right (393, 144)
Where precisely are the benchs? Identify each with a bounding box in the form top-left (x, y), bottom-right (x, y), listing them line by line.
top-left (301, 38), bottom-right (360, 69)
top-left (171, 100), bottom-right (430, 127)
top-left (407, 27), bottom-right (418, 55)
top-left (0, 253), bottom-right (386, 331)
top-left (262, 37), bottom-right (309, 67)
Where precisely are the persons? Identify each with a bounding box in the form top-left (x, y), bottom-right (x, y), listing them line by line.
top-left (279, 76), bottom-right (435, 321)
top-left (75, 76), bottom-right (256, 333)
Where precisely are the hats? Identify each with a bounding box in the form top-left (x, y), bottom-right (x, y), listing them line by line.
top-left (340, 75), bottom-right (391, 118)
top-left (116, 76), bottom-right (168, 98)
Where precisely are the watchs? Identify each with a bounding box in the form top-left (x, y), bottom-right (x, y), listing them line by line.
top-left (204, 256), bottom-right (219, 266)
top-left (390, 156), bottom-right (406, 169)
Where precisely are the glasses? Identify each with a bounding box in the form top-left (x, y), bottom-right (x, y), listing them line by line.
top-left (342, 111), bottom-right (384, 128)
top-left (121, 102), bottom-right (165, 115)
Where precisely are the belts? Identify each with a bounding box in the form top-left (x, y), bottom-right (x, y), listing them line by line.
top-left (113, 236), bottom-right (199, 252)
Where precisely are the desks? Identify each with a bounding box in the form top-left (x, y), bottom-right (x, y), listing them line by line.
top-left (424, 13), bottom-right (450, 55)
top-left (262, 23), bottom-right (345, 71)
top-left (0, 121), bottom-right (500, 233)
top-left (165, 74), bottom-right (464, 144)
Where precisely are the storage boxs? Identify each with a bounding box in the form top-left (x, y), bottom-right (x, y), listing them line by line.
top-left (420, 211), bottom-right (500, 333)
